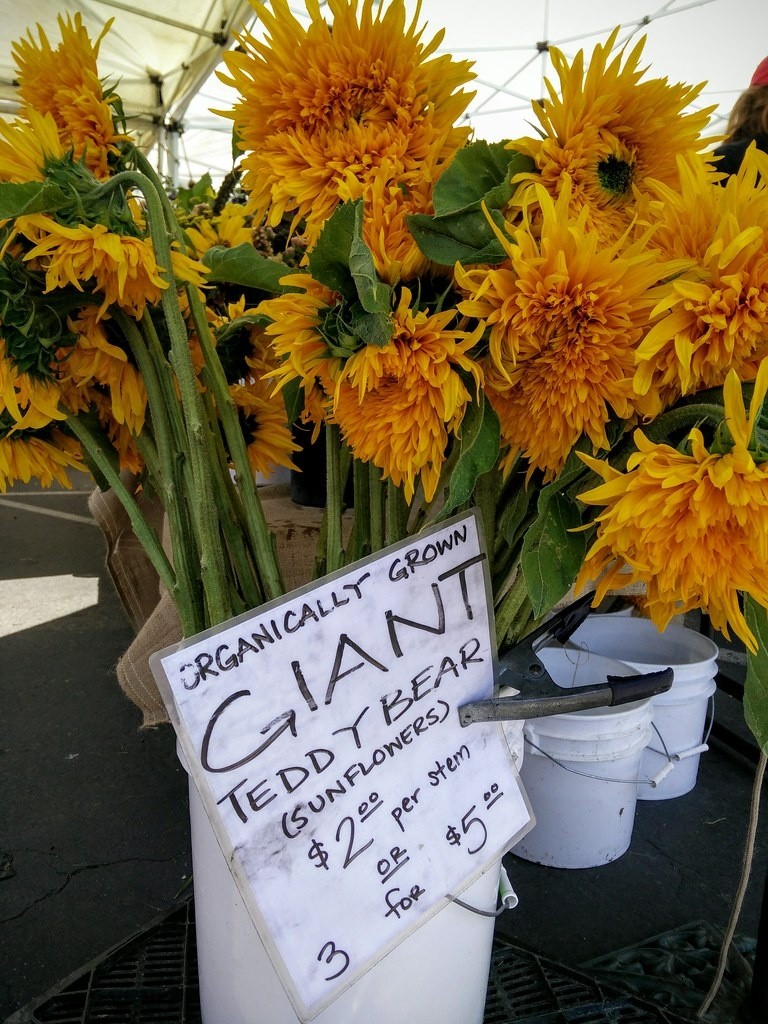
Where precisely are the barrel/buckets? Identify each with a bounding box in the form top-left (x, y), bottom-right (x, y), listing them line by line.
top-left (509, 648), bottom-right (674, 868)
top-left (561, 617), bottom-right (718, 800)
top-left (174, 745), bottom-right (517, 1024)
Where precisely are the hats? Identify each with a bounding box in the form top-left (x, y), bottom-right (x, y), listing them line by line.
top-left (751, 56), bottom-right (768, 85)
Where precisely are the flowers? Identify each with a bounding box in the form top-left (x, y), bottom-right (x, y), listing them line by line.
top-left (1, 0), bottom-right (767, 662)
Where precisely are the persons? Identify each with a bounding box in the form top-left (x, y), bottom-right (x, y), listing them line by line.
top-left (705, 55), bottom-right (768, 189)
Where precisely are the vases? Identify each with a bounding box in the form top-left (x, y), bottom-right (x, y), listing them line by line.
top-left (288, 419), bottom-right (356, 508)
